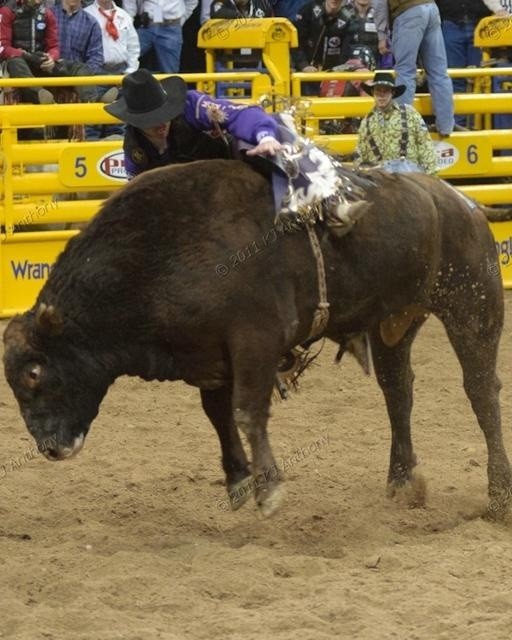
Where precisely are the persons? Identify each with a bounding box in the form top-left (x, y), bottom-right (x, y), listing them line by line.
top-left (100, 66), bottom-right (375, 237)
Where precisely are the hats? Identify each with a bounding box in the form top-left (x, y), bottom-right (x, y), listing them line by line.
top-left (102, 69), bottom-right (189, 129)
top-left (359, 70), bottom-right (407, 101)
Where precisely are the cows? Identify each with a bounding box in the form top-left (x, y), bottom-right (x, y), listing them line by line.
top-left (0, 154), bottom-right (511, 515)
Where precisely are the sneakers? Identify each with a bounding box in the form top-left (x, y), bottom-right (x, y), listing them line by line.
top-left (38, 88), bottom-right (57, 104)
top-left (99, 87), bottom-right (119, 103)
top-left (328, 199), bottom-right (368, 238)
